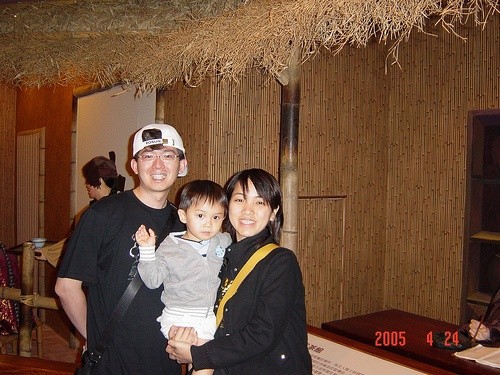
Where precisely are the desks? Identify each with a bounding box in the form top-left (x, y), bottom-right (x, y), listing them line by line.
top-left (321, 308), bottom-right (500, 375)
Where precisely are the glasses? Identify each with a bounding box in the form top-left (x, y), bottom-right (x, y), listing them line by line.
top-left (136, 154), bottom-right (179, 161)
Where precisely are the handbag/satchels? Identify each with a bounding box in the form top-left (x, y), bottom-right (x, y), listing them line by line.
top-left (81, 349), bottom-right (101, 375)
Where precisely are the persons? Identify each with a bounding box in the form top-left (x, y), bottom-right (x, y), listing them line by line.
top-left (165, 168), bottom-right (312, 375)
top-left (35, 155), bottom-right (127, 269)
top-left (54, 123), bottom-right (188, 375)
top-left (134, 180), bottom-right (233, 375)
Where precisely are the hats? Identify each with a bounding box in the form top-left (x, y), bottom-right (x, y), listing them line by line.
top-left (133, 124), bottom-right (187, 177)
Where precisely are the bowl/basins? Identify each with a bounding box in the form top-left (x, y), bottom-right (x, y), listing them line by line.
top-left (31, 238), bottom-right (47, 247)
